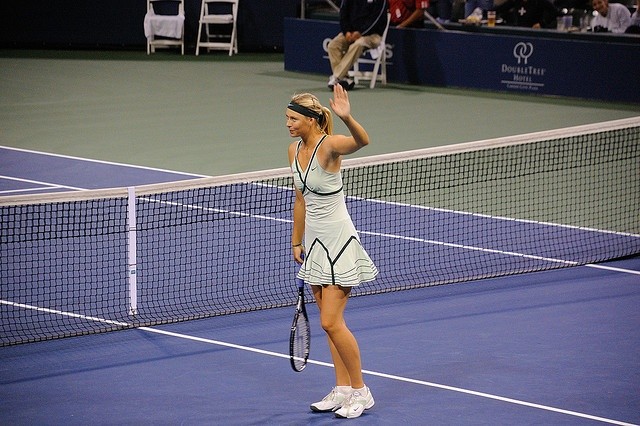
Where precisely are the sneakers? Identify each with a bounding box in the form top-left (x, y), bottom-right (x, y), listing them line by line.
top-left (328, 76), bottom-right (338, 88)
top-left (339, 77), bottom-right (355, 89)
top-left (335, 387), bottom-right (375, 419)
top-left (310, 387), bottom-right (351, 412)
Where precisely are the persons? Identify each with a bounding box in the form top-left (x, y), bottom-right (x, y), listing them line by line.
top-left (590, 0), bottom-right (630, 33)
top-left (390, 0), bottom-right (429, 28)
top-left (625, 0), bottom-right (640, 33)
top-left (327, 0), bottom-right (388, 90)
top-left (431, 0), bottom-right (557, 29)
top-left (283, 82), bottom-right (380, 421)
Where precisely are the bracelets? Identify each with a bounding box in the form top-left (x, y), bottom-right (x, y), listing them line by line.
top-left (292, 243), bottom-right (302, 247)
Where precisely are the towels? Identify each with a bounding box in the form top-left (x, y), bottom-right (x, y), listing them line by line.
top-left (143, 9), bottom-right (185, 38)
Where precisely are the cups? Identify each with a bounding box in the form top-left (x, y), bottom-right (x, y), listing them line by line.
top-left (487, 9), bottom-right (496, 27)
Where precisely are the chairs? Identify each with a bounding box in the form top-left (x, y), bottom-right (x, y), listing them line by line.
top-left (347, 12), bottom-right (392, 89)
top-left (145, 0), bottom-right (185, 55)
top-left (195, 0), bottom-right (239, 56)
